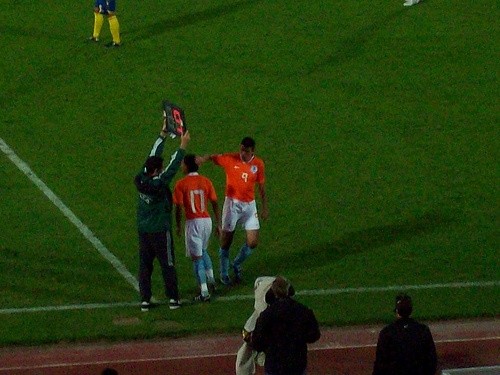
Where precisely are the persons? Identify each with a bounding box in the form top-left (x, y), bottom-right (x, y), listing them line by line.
top-left (242, 275), bottom-right (321, 375)
top-left (87, 0), bottom-right (122, 46)
top-left (132, 118), bottom-right (191, 312)
top-left (196, 136), bottom-right (269, 286)
top-left (173, 153), bottom-right (223, 303)
top-left (371, 293), bottom-right (444, 375)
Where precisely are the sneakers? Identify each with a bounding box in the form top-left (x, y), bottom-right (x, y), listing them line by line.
top-left (220, 272), bottom-right (233, 286)
top-left (195, 284), bottom-right (217, 295)
top-left (231, 259), bottom-right (242, 278)
top-left (141, 302), bottom-right (151, 312)
top-left (192, 294), bottom-right (211, 303)
top-left (169, 298), bottom-right (182, 309)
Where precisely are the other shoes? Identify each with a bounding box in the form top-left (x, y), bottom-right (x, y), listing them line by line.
top-left (103, 41), bottom-right (121, 47)
top-left (403, 0), bottom-right (418, 6)
top-left (85, 36), bottom-right (100, 42)
